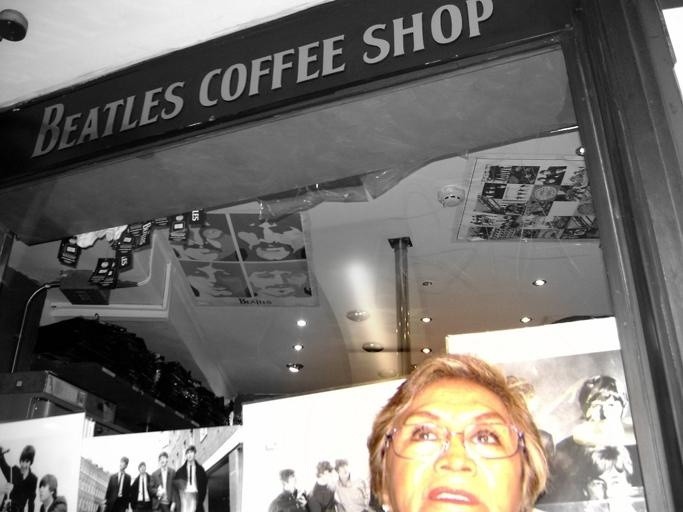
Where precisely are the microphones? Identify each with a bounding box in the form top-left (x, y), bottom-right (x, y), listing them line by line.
top-left (11, 280), bottom-right (62, 373)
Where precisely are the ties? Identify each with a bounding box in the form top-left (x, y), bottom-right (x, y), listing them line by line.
top-left (117, 473), bottom-right (123, 495)
top-left (189, 465), bottom-right (193, 485)
top-left (142, 477), bottom-right (146, 502)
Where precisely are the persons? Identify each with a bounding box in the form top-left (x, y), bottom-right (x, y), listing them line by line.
top-left (466, 160), bottom-right (600, 242)
top-left (367, 354), bottom-right (548, 512)
top-left (526, 376), bottom-right (648, 512)
top-left (102, 445), bottom-right (209, 512)
top-left (268, 459), bottom-right (365, 511)
top-left (38, 474), bottom-right (67, 512)
top-left (1, 445), bottom-right (37, 512)
top-left (167, 211), bottom-right (312, 299)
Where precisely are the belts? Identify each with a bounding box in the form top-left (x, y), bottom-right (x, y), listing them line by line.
top-left (137, 501), bottom-right (151, 505)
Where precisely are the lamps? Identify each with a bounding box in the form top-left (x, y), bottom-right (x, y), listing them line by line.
top-left (285, 342), bottom-right (307, 373)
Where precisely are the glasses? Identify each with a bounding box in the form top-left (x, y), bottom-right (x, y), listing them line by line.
top-left (385, 422), bottom-right (528, 461)
